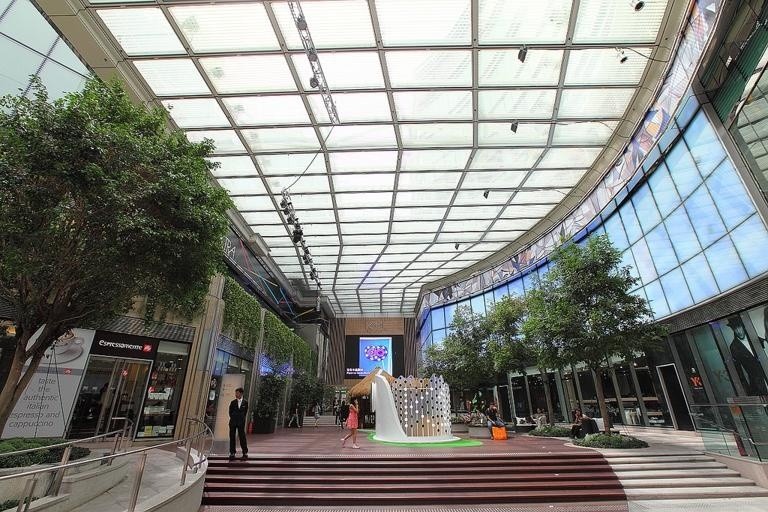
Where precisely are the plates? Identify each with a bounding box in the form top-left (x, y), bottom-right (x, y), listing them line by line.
top-left (36, 344), bottom-right (83, 365)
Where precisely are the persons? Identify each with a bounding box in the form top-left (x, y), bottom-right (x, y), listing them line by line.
top-left (571, 408), bottom-right (587, 439)
top-left (332, 396), bottom-right (360, 448)
top-left (726, 317), bottom-right (768, 397)
top-left (229, 387), bottom-right (252, 460)
top-left (312, 401), bottom-right (321, 428)
top-left (288, 402), bottom-right (302, 428)
top-left (487, 400), bottom-right (505, 439)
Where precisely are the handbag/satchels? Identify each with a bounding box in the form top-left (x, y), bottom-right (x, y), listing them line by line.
top-left (492, 426), bottom-right (507, 440)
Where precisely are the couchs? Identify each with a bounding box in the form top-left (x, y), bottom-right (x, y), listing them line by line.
top-left (588, 417), bottom-right (620, 440)
top-left (570, 419), bottom-right (589, 439)
top-left (511, 415), bottom-right (549, 434)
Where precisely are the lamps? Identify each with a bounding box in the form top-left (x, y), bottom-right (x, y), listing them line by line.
top-left (516, 41), bottom-right (528, 64)
top-left (296, 13), bottom-right (318, 88)
top-left (454, 242), bottom-right (460, 250)
top-left (611, 43), bottom-right (670, 65)
top-left (509, 117), bottom-right (519, 135)
top-left (279, 189), bottom-right (322, 290)
top-left (481, 188), bottom-right (489, 200)
top-left (625, 0), bottom-right (665, 11)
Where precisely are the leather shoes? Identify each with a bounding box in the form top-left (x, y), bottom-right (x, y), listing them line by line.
top-left (229, 453), bottom-right (248, 457)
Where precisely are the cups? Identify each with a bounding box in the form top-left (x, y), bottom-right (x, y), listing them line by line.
top-left (46, 331), bottom-right (85, 356)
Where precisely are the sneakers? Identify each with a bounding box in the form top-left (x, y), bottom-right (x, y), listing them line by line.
top-left (341, 439), bottom-right (360, 449)
top-left (491, 436), bottom-right (494, 440)
